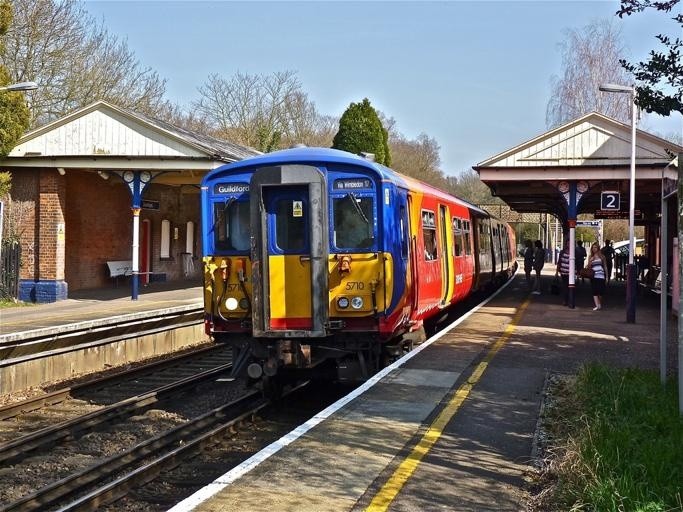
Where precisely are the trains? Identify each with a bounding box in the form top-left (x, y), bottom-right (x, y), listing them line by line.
top-left (203, 141), bottom-right (519, 402)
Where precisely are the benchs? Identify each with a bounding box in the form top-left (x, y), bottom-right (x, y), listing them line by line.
top-left (106, 261), bottom-right (154, 288)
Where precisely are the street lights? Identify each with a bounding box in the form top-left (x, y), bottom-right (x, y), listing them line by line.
top-left (598, 84), bottom-right (638, 265)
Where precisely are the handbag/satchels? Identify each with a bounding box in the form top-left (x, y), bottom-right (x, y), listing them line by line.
top-left (580, 268), bottom-right (594, 279)
top-left (551, 279), bottom-right (561, 296)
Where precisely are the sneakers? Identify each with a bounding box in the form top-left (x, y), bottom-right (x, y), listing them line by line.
top-left (593, 304), bottom-right (602, 311)
top-left (522, 277), bottom-right (530, 281)
top-left (532, 290), bottom-right (541, 295)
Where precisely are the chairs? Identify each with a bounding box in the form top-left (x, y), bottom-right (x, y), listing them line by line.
top-left (636, 264), bottom-right (661, 309)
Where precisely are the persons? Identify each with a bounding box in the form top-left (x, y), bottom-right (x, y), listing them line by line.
top-left (519, 239), bottom-right (534, 292)
top-left (555, 239), bottom-right (616, 312)
top-left (531, 240), bottom-right (546, 295)
